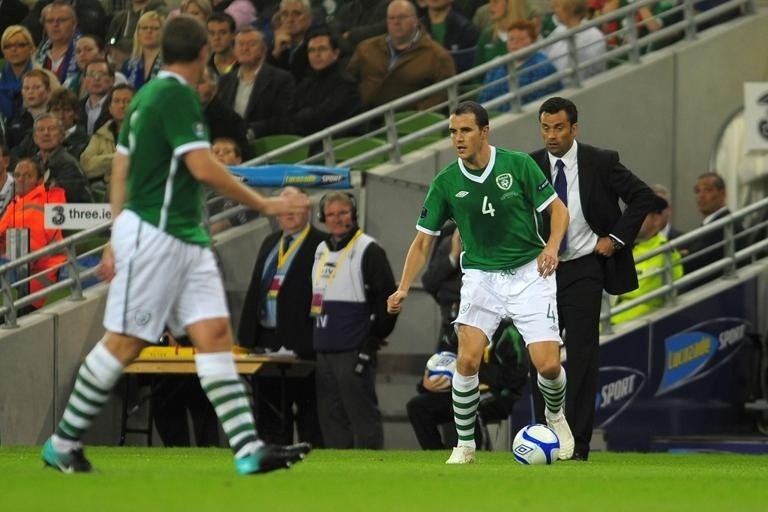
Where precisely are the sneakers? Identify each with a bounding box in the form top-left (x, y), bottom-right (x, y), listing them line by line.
top-left (41, 437), bottom-right (91, 472)
top-left (234, 435), bottom-right (310, 475)
top-left (546, 416), bottom-right (587, 460)
top-left (446, 446), bottom-right (475, 464)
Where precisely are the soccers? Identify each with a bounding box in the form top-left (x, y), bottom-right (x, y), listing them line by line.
top-left (425, 352), bottom-right (458, 391)
top-left (512, 424), bottom-right (560, 465)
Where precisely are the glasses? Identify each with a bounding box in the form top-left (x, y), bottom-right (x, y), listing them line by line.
top-left (87, 71), bottom-right (108, 77)
top-left (4, 42), bottom-right (28, 49)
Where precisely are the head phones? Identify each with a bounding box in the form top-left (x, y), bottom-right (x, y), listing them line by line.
top-left (316, 191), bottom-right (358, 224)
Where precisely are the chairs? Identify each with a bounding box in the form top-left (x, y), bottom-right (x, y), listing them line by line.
top-left (323, 137), bottom-right (387, 172)
top-left (386, 131), bottom-right (441, 159)
top-left (250, 133), bottom-right (307, 170)
top-left (395, 110), bottom-right (450, 134)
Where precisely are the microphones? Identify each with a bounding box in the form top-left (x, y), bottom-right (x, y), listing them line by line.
top-left (345, 223), bottom-right (356, 229)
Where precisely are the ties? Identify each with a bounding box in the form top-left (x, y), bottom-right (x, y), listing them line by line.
top-left (554, 160), bottom-right (567, 257)
top-left (261, 235), bottom-right (293, 309)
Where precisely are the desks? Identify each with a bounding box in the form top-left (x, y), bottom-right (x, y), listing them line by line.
top-left (118, 347), bottom-right (317, 450)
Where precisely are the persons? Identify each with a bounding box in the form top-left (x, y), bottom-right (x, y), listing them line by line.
top-left (236, 186), bottom-right (332, 452)
top-left (599, 196), bottom-right (684, 333)
top-left (151, 323), bottom-right (221, 447)
top-left (39, 13), bottom-right (311, 478)
top-left (524, 95), bottom-right (656, 462)
top-left (406, 326), bottom-right (516, 452)
top-left (385, 99), bottom-right (576, 467)
top-left (309, 189), bottom-right (402, 449)
top-left (1, 1), bottom-right (747, 327)
